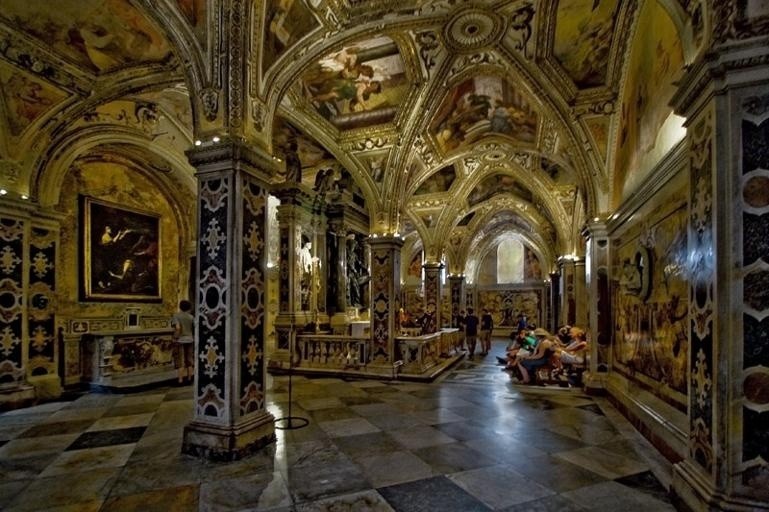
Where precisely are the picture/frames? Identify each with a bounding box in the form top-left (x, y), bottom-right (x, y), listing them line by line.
top-left (77, 195), bottom-right (164, 303)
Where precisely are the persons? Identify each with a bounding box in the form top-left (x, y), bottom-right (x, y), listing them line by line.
top-left (457, 309), bottom-right (466, 350)
top-left (480, 308), bottom-right (491, 358)
top-left (462, 307), bottom-right (479, 358)
top-left (97, 225), bottom-right (130, 289)
top-left (303, 43), bottom-right (383, 112)
top-left (347, 238), bottom-right (369, 303)
top-left (300, 240), bottom-right (321, 304)
top-left (131, 230), bottom-right (158, 294)
top-left (496, 310), bottom-right (588, 385)
top-left (486, 312), bottom-right (493, 352)
top-left (170, 300), bottom-right (196, 386)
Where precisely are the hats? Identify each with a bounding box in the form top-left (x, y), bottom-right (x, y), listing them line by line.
top-left (528, 327), bottom-right (550, 337)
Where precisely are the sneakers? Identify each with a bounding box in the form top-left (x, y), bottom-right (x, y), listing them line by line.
top-left (495, 355), bottom-right (531, 385)
top-left (457, 348), bottom-right (492, 360)
